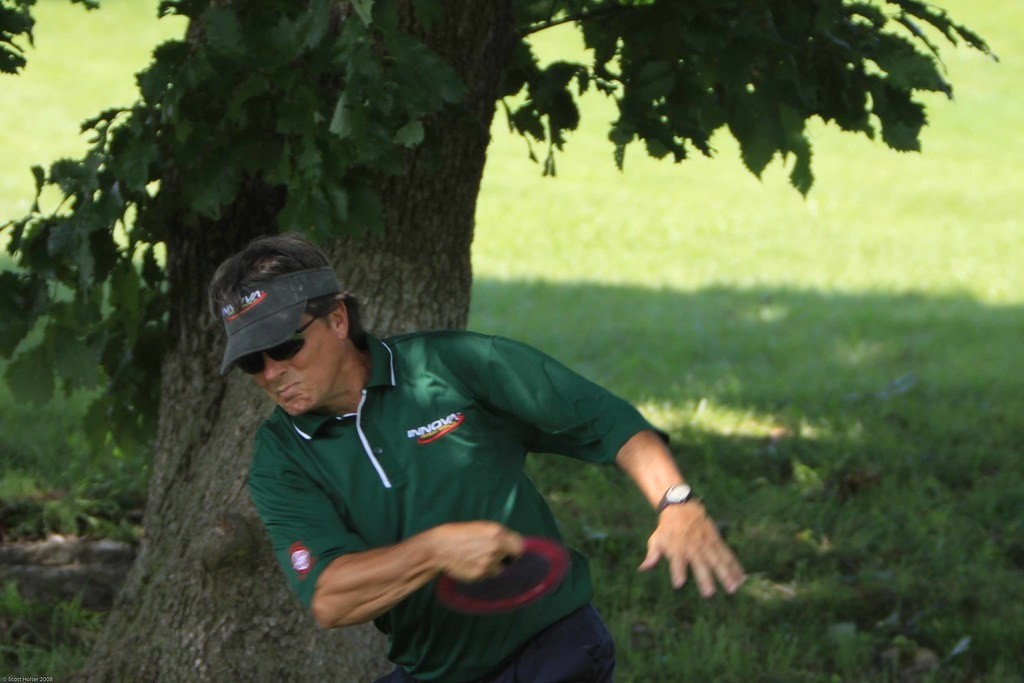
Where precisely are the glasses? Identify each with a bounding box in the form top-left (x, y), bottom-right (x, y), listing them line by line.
top-left (236, 313), bottom-right (322, 375)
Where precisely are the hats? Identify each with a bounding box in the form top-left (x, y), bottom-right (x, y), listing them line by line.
top-left (220, 267), bottom-right (339, 376)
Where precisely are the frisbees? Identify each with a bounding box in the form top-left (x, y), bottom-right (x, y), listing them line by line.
top-left (438, 537), bottom-right (566, 610)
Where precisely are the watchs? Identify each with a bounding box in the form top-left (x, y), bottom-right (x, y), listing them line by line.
top-left (656, 482), bottom-right (695, 518)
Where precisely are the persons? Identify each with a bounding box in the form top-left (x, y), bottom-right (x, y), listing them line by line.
top-left (210, 236), bottom-right (747, 682)
